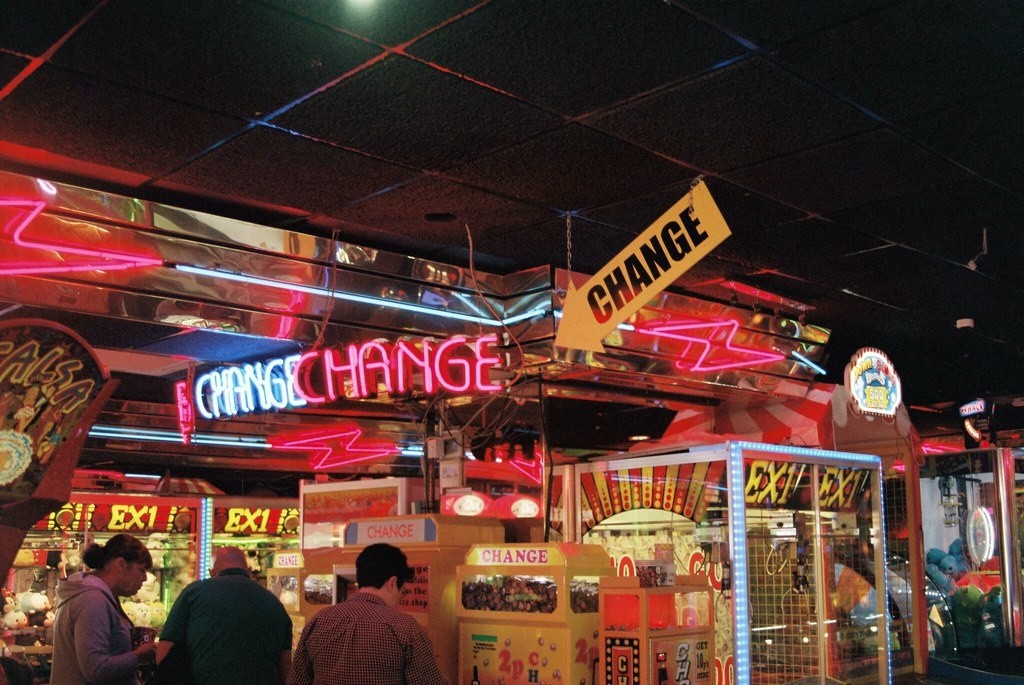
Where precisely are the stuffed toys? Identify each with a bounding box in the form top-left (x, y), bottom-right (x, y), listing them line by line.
top-left (927, 537), bottom-right (1008, 650)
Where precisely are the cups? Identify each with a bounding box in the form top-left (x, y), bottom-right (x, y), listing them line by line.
top-left (634, 543), bottom-right (677, 587)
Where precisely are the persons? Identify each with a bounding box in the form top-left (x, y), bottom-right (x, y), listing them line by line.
top-left (294, 541), bottom-right (449, 684)
top-left (160, 547), bottom-right (293, 685)
top-left (50, 533), bottom-right (158, 685)
